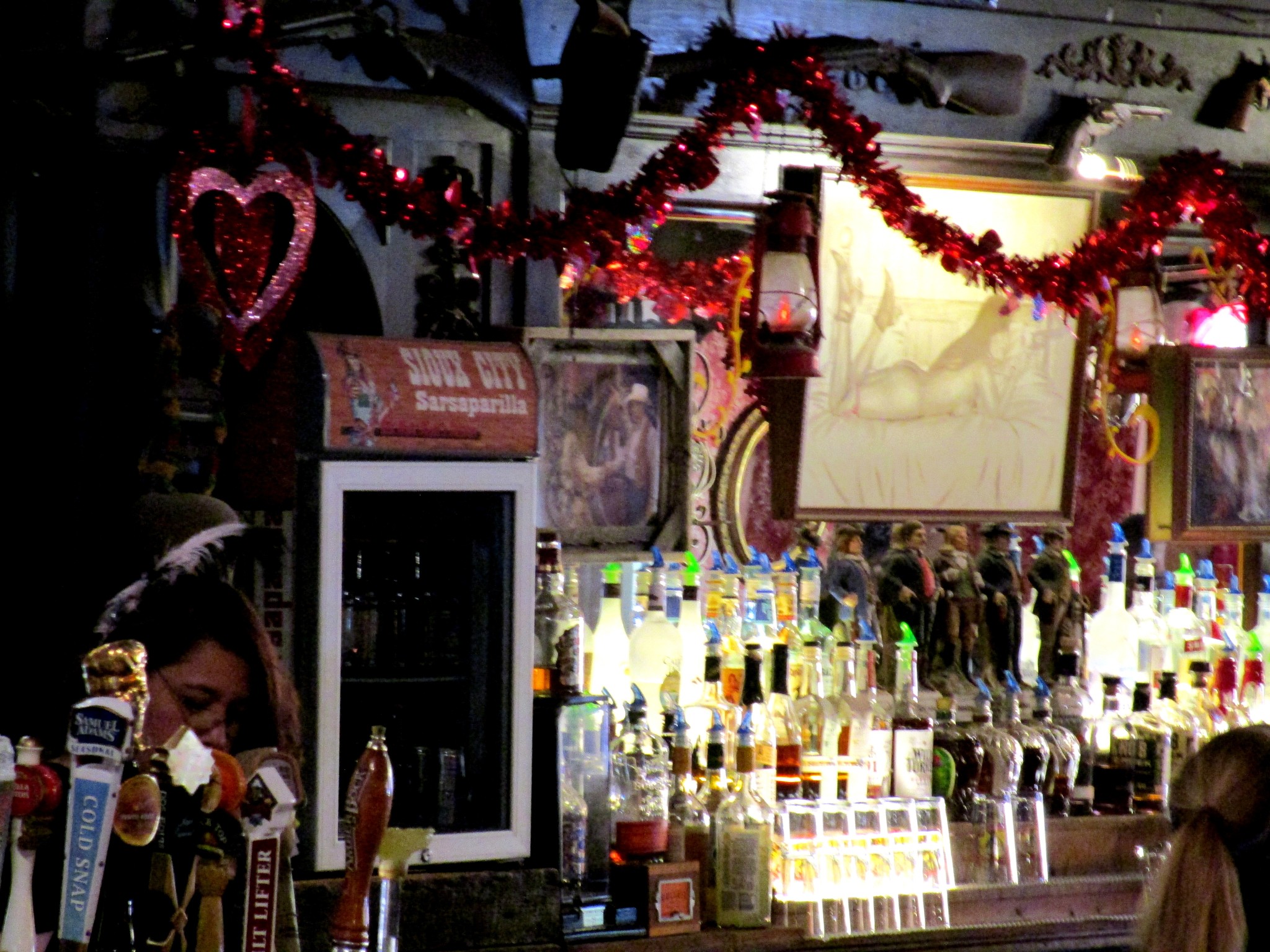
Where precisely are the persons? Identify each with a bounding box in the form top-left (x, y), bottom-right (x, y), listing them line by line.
top-left (792, 508), bottom-right (1087, 690)
top-left (64, 566), bottom-right (302, 757)
top-left (1132, 724), bottom-right (1270, 952)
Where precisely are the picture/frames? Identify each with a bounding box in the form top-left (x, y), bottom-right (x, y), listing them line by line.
top-left (788, 163), bottom-right (1102, 527)
top-left (1171, 345), bottom-right (1269, 545)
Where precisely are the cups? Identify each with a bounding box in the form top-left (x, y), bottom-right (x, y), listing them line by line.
top-left (1133, 842), bottom-right (1171, 910)
top-left (969, 792), bottom-right (1048, 887)
top-left (362, 876), bottom-right (401, 952)
top-left (850, 798), bottom-right (955, 893)
top-left (785, 842), bottom-right (853, 939)
top-left (772, 796), bottom-right (853, 901)
top-left (853, 831), bottom-right (951, 933)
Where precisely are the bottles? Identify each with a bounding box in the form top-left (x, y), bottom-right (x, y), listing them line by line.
top-left (1006, 522), bottom-right (1170, 717)
top-left (610, 682), bottom-right (677, 862)
top-left (1157, 553), bottom-right (1270, 701)
top-left (341, 546), bottom-right (435, 677)
top-left (692, 621), bottom-right (803, 800)
top-left (714, 714), bottom-right (772, 928)
top-left (533, 528), bottom-right (594, 697)
top-left (683, 549), bottom-right (723, 706)
top-left (558, 692), bottom-right (616, 884)
top-left (965, 652), bottom-right (1097, 818)
top-left (594, 546), bottom-right (682, 707)
top-left (773, 548), bottom-right (833, 694)
top-left (793, 639), bottom-right (871, 802)
top-left (676, 706), bottom-right (732, 928)
top-left (1087, 630), bottom-right (1270, 815)
top-left (935, 697), bottom-right (984, 821)
top-left (858, 621), bottom-right (935, 800)
top-left (723, 546), bottom-right (777, 702)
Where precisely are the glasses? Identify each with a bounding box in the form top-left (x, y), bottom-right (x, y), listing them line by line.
top-left (154, 668), bottom-right (249, 732)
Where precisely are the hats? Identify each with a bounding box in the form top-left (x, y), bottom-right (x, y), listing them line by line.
top-left (625, 383), bottom-right (653, 407)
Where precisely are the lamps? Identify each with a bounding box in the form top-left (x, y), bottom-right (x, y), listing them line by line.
top-left (743, 114), bottom-right (826, 382)
